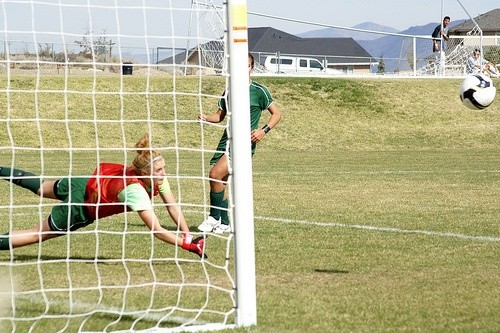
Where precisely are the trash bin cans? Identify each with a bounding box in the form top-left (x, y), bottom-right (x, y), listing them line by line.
top-left (123, 62), bottom-right (133, 75)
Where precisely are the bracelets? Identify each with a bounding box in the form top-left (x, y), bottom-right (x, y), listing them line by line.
top-left (262, 125), bottom-right (271, 134)
top-left (443, 34), bottom-right (446, 36)
top-left (435, 44), bottom-right (438, 46)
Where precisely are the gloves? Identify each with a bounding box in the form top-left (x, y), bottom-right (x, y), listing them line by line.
top-left (182, 234), bottom-right (209, 260)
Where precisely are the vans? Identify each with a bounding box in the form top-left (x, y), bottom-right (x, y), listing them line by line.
top-left (264, 56), bottom-right (326, 76)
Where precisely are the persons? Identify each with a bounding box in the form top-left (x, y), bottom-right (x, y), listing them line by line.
top-left (467, 49), bottom-right (500, 77)
top-left (432, 16), bottom-right (451, 54)
top-left (0, 134), bottom-right (207, 258)
top-left (197, 53), bottom-right (281, 234)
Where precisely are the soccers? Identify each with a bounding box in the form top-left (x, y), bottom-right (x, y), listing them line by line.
top-left (458, 73), bottom-right (496, 110)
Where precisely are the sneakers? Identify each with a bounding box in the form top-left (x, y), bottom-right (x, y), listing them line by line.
top-left (198, 214), bottom-right (222, 232)
top-left (212, 223), bottom-right (231, 234)
